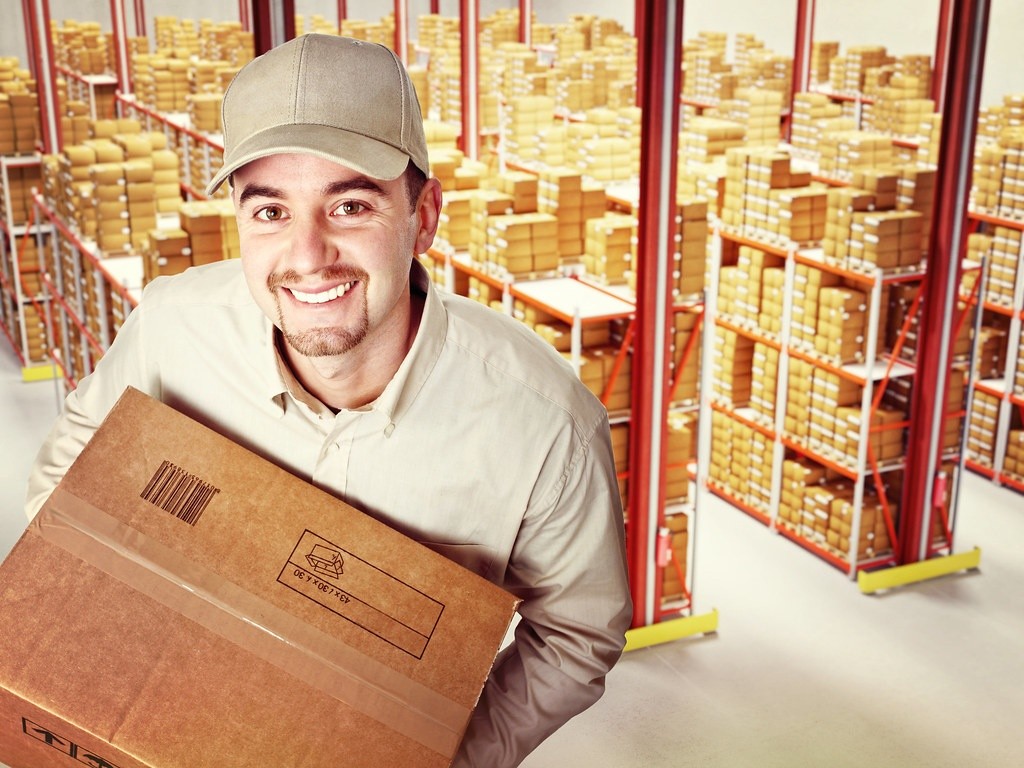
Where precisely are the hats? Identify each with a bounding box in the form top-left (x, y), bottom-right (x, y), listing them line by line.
top-left (205, 32), bottom-right (429, 196)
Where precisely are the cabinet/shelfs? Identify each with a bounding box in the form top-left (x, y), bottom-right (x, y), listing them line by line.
top-left (1, 0), bottom-right (1024, 653)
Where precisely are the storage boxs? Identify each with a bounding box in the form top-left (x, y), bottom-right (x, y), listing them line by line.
top-left (0, 384), bottom-right (526, 767)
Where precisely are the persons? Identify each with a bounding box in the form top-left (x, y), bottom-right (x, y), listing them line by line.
top-left (17, 35), bottom-right (633, 768)
